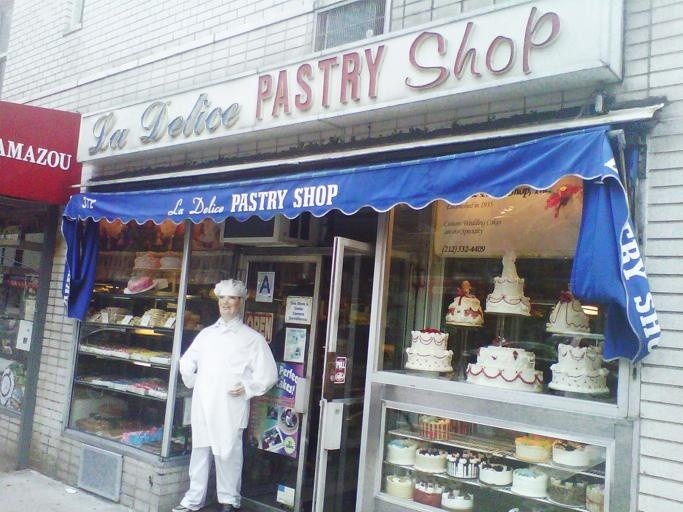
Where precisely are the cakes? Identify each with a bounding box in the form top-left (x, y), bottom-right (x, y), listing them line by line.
top-left (75, 252), bottom-right (200, 447)
top-left (548, 343), bottom-right (609, 394)
top-left (445, 280), bottom-right (485, 327)
top-left (544, 290), bottom-right (591, 334)
top-left (405, 328), bottom-right (454, 372)
top-left (466, 345), bottom-right (543, 393)
top-left (484, 255), bottom-right (532, 317)
top-left (386, 416), bottom-right (605, 512)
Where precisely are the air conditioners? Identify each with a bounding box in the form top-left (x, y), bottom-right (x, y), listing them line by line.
top-left (218, 212), bottom-right (303, 251)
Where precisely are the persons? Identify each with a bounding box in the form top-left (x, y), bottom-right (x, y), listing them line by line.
top-left (171, 278), bottom-right (279, 512)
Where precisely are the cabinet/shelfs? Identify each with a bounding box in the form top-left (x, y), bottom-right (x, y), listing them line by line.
top-left (62, 221), bottom-right (236, 458)
top-left (349, 182), bottom-right (626, 510)
